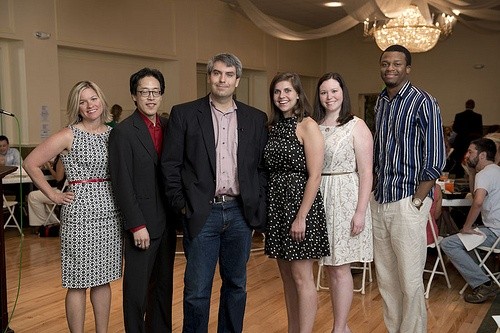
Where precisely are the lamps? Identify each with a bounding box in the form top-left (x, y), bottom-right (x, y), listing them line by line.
top-left (229, 0), bottom-right (500, 53)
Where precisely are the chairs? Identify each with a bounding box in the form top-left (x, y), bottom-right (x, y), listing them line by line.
top-left (458, 235), bottom-right (500, 295)
top-left (423, 211), bottom-right (451, 298)
top-left (39, 179), bottom-right (68, 235)
top-left (3, 194), bottom-right (25, 237)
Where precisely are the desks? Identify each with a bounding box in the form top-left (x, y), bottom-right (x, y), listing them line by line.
top-left (0, 165), bottom-right (50, 234)
top-left (441, 199), bottom-right (472, 234)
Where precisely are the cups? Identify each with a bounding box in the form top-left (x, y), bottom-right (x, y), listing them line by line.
top-left (445, 179), bottom-right (454, 192)
top-left (440, 172), bottom-right (448, 180)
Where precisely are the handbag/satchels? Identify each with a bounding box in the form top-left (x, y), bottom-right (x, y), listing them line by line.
top-left (39, 224), bottom-right (60, 237)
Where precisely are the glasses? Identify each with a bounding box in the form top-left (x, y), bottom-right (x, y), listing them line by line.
top-left (137, 90), bottom-right (162, 97)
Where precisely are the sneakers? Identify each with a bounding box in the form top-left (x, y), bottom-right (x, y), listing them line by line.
top-left (464, 281), bottom-right (499, 303)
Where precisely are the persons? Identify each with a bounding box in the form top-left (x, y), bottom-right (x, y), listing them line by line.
top-left (483, 133), bottom-right (500, 167)
top-left (108, 67), bottom-right (183, 333)
top-left (0, 135), bottom-right (23, 166)
top-left (440, 138), bottom-right (500, 303)
top-left (27, 154), bottom-right (68, 237)
top-left (158, 54), bottom-right (269, 333)
top-left (262, 72), bottom-right (332, 333)
top-left (312, 72), bottom-right (375, 333)
top-left (105, 104), bottom-right (122, 127)
top-left (22, 81), bottom-right (124, 333)
top-left (423, 184), bottom-right (456, 280)
top-left (453, 99), bottom-right (483, 178)
top-left (370, 45), bottom-right (446, 333)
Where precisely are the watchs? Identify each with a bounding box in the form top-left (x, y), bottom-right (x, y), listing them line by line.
top-left (412, 196), bottom-right (423, 209)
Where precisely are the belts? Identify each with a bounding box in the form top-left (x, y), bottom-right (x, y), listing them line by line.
top-left (321, 172), bottom-right (353, 176)
top-left (69, 179), bottom-right (110, 184)
top-left (213, 194), bottom-right (238, 203)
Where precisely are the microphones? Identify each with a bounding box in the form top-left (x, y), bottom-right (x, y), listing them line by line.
top-left (0, 109), bottom-right (14, 117)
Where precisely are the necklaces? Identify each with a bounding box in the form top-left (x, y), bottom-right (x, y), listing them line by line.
top-left (82, 121), bottom-right (102, 132)
top-left (324, 117), bottom-right (336, 132)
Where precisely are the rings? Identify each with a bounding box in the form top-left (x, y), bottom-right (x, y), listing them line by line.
top-left (360, 230), bottom-right (363, 232)
top-left (138, 244), bottom-right (140, 246)
